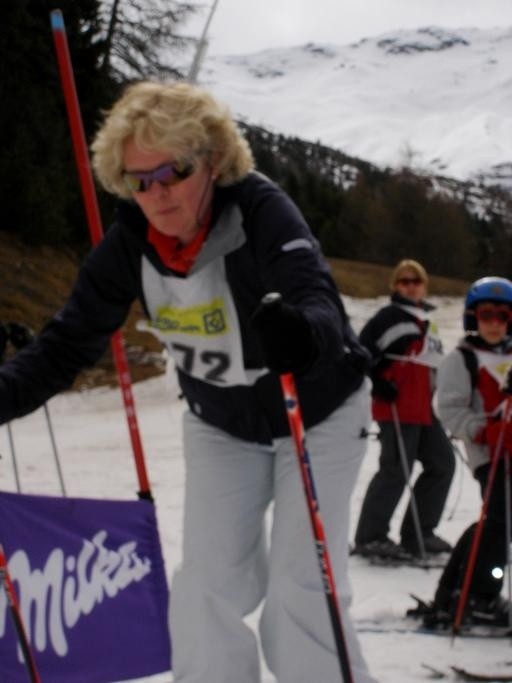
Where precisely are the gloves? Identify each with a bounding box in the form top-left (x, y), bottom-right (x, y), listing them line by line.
top-left (253, 300), bottom-right (314, 375)
top-left (474, 421), bottom-right (511, 448)
top-left (368, 378), bottom-right (398, 404)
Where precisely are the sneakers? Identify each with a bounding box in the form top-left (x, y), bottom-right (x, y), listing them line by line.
top-left (426, 592), bottom-right (511, 634)
top-left (400, 531), bottom-right (454, 556)
top-left (357, 537), bottom-right (415, 562)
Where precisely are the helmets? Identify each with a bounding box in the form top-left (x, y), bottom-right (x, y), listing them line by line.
top-left (462, 276), bottom-right (511, 331)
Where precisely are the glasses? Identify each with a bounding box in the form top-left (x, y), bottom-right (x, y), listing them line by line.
top-left (476, 305), bottom-right (511, 324)
top-left (395, 276), bottom-right (423, 286)
top-left (119, 152), bottom-right (206, 195)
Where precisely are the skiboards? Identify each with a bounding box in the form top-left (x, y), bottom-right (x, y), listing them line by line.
top-left (349, 546), bottom-right (453, 568)
top-left (421, 663), bottom-right (511, 682)
top-left (352, 614), bottom-right (512, 638)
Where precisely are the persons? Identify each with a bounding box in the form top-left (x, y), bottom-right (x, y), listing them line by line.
top-left (345, 255), bottom-right (457, 563)
top-left (413, 271), bottom-right (510, 636)
top-left (0, 77), bottom-right (378, 682)
